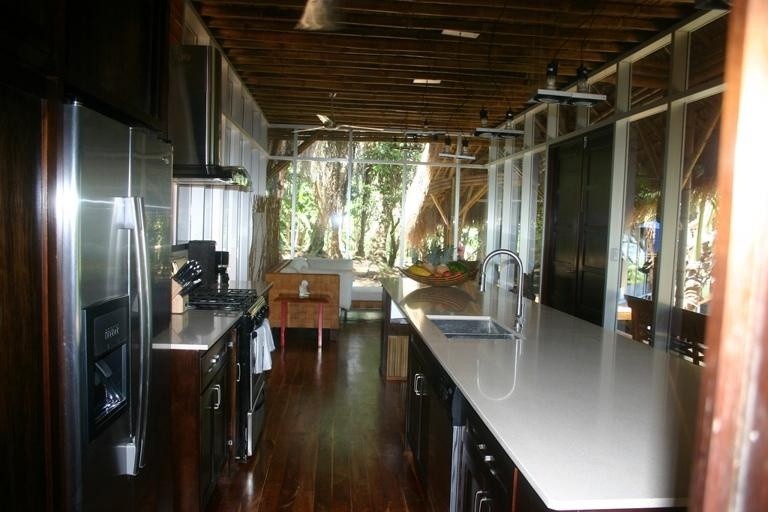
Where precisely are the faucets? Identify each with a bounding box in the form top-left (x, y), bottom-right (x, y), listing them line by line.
top-left (475, 337), bottom-right (520, 402)
top-left (480, 249), bottom-right (524, 318)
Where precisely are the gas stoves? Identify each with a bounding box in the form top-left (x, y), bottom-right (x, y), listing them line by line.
top-left (191, 289), bottom-right (260, 313)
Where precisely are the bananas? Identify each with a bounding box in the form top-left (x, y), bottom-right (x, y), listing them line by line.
top-left (408, 266), bottom-right (431, 277)
top-left (408, 302), bottom-right (432, 309)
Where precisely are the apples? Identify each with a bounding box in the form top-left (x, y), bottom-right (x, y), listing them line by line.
top-left (443, 271), bottom-right (452, 280)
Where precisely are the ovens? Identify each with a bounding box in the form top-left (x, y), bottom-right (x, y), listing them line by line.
top-left (239, 300), bottom-right (271, 463)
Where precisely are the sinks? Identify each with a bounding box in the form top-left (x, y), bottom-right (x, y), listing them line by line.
top-left (427, 313), bottom-right (523, 341)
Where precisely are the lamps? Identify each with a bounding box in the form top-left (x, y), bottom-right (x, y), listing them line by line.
top-left (392, 0), bottom-right (607, 160)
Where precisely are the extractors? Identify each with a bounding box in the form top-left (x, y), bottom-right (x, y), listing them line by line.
top-left (174, 44), bottom-right (254, 192)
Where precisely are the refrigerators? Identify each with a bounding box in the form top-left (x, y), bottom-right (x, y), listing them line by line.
top-left (54, 99), bottom-right (179, 512)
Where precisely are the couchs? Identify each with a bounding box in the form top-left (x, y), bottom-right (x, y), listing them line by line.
top-left (263, 259), bottom-right (352, 341)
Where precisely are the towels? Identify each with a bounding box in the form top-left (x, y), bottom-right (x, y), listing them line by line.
top-left (251, 318), bottom-right (276, 375)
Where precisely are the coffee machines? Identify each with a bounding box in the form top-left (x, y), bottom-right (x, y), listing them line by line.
top-left (215, 250), bottom-right (230, 284)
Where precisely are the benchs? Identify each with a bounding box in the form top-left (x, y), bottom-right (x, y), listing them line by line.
top-left (623, 295), bottom-right (708, 367)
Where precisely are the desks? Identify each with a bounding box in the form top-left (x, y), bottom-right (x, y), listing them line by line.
top-left (272, 292), bottom-right (330, 347)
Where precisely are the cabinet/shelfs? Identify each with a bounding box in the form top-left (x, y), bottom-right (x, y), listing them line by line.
top-left (0, 0), bottom-right (173, 145)
top-left (405, 324), bottom-right (456, 511)
top-left (150, 317), bottom-right (252, 512)
top-left (457, 445), bottom-right (498, 512)
top-left (218, 46), bottom-right (269, 195)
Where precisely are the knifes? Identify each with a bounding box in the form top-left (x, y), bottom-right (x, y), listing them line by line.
top-left (176, 258), bottom-right (201, 296)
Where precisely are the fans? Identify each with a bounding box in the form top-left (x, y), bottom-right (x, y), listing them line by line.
top-left (289, 90), bottom-right (385, 134)
